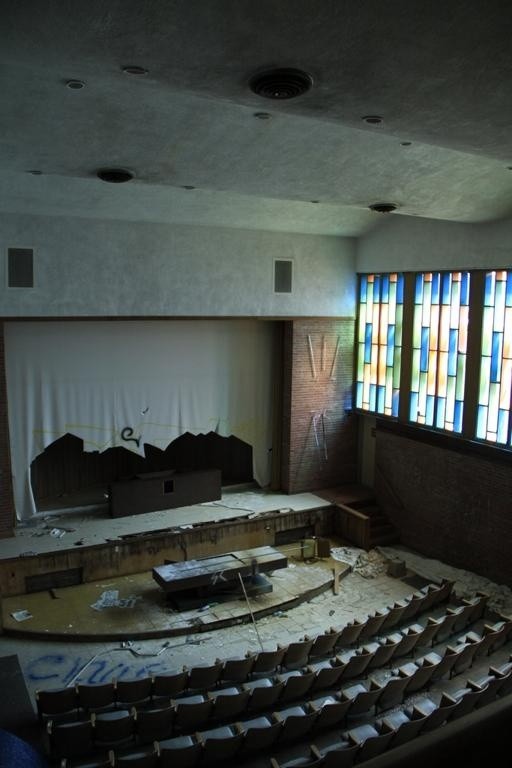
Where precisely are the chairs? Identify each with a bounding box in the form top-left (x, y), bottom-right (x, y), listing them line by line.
top-left (291, 534), bottom-right (331, 565)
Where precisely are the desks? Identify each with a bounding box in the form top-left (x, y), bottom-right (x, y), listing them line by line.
top-left (153, 544), bottom-right (291, 612)
